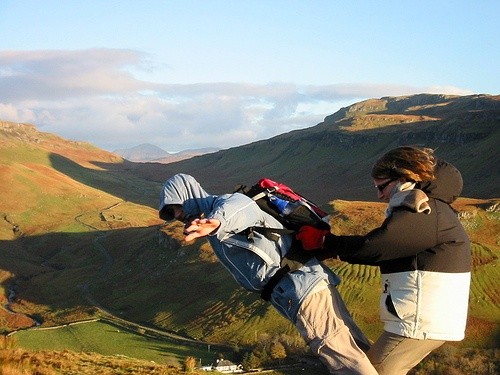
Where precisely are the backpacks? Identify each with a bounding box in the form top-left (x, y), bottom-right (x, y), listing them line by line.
top-left (211, 178), bottom-right (331, 235)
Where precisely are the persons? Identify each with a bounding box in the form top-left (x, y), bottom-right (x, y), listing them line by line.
top-left (294, 146), bottom-right (473, 375)
top-left (157, 173), bottom-right (381, 375)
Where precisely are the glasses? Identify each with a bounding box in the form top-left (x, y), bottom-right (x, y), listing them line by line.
top-left (375, 179), bottom-right (398, 193)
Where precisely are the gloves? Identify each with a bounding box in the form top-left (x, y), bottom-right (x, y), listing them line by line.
top-left (295, 225), bottom-right (328, 250)
